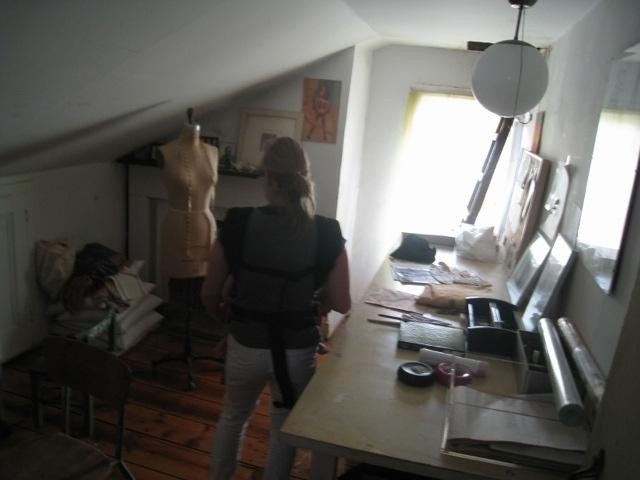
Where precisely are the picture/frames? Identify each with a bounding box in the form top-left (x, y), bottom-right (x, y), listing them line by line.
top-left (522, 234), bottom-right (576, 333)
top-left (494, 149), bottom-right (552, 274)
top-left (507, 231), bottom-right (553, 305)
top-left (234, 109), bottom-right (303, 172)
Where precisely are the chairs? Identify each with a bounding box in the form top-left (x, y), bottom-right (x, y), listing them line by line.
top-left (0, 334), bottom-right (136, 480)
top-left (29, 306), bottom-right (116, 435)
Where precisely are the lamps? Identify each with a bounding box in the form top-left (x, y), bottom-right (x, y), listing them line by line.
top-left (472, 1), bottom-right (548, 117)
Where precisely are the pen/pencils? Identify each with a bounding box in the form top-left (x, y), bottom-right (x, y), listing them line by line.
top-left (367, 318), bottom-right (400, 325)
top-left (379, 313), bottom-right (405, 321)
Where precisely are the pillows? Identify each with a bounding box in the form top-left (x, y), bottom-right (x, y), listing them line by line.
top-left (55, 282), bottom-right (166, 357)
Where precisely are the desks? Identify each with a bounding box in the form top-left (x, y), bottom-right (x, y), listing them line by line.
top-left (114, 141), bottom-right (267, 306)
top-left (279, 231), bottom-right (594, 478)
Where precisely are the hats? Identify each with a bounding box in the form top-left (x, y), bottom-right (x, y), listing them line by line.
top-left (391, 236), bottom-right (436, 264)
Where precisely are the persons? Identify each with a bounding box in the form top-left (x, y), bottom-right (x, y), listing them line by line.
top-left (158, 121), bottom-right (219, 279)
top-left (202, 136), bottom-right (351, 480)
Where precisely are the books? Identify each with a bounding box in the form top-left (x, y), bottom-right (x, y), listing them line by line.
top-left (399, 319), bottom-right (467, 358)
